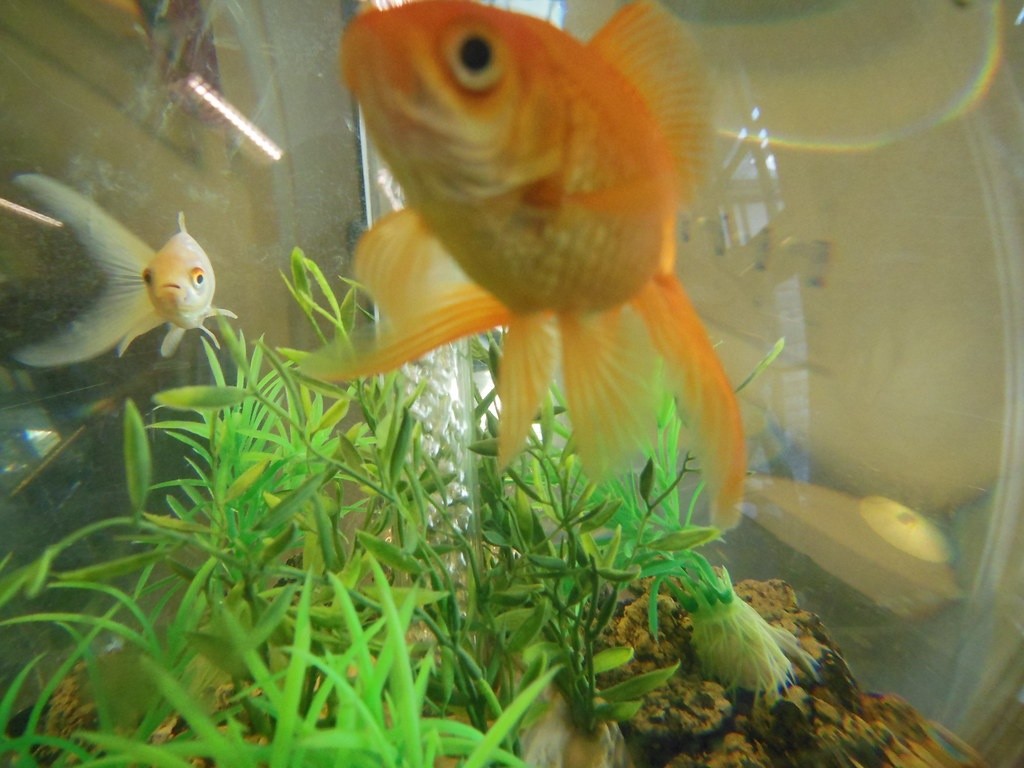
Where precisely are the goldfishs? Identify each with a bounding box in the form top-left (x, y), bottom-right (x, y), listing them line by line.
top-left (288, 0), bottom-right (751, 534)
top-left (9, 171), bottom-right (240, 367)
